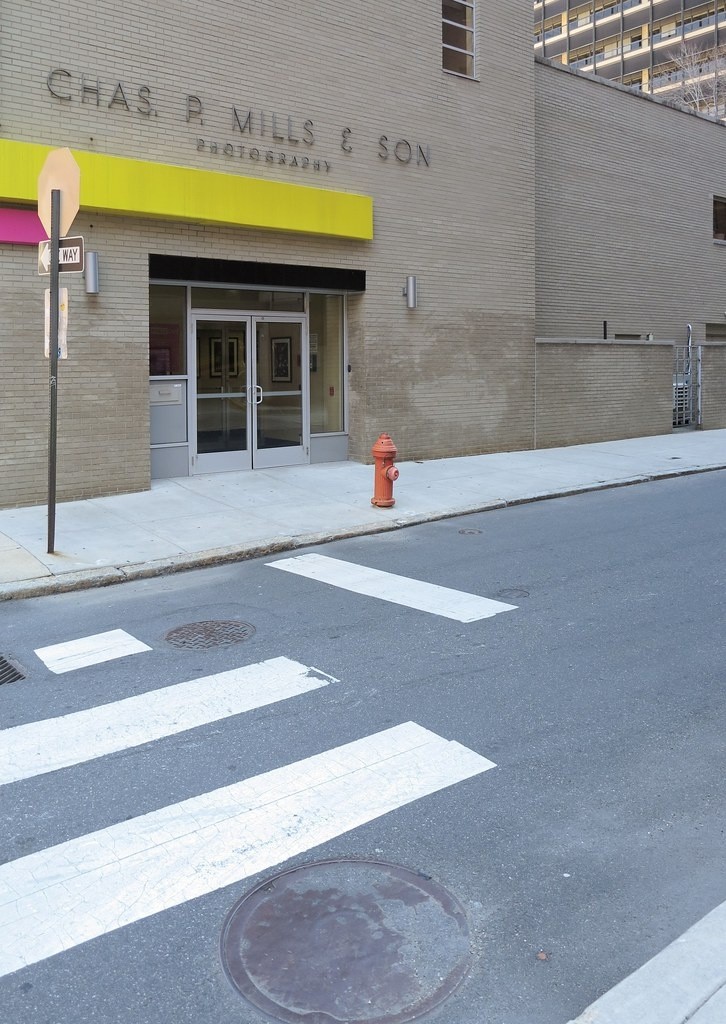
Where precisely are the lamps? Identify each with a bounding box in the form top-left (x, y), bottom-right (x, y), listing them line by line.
top-left (83, 251), bottom-right (100, 294)
top-left (403, 276), bottom-right (418, 308)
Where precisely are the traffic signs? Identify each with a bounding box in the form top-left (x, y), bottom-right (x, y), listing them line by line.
top-left (37, 236), bottom-right (84, 276)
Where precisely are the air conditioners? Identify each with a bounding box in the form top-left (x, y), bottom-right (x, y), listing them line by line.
top-left (673, 382), bottom-right (688, 410)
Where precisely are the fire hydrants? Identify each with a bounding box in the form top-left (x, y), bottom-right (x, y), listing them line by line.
top-left (370, 432), bottom-right (399, 507)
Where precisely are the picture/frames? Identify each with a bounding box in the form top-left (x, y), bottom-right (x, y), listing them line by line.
top-left (197, 337), bottom-right (200, 379)
top-left (208, 337), bottom-right (239, 378)
top-left (270, 336), bottom-right (292, 382)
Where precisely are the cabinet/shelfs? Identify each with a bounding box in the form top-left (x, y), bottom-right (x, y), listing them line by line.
top-left (150, 347), bottom-right (172, 375)
